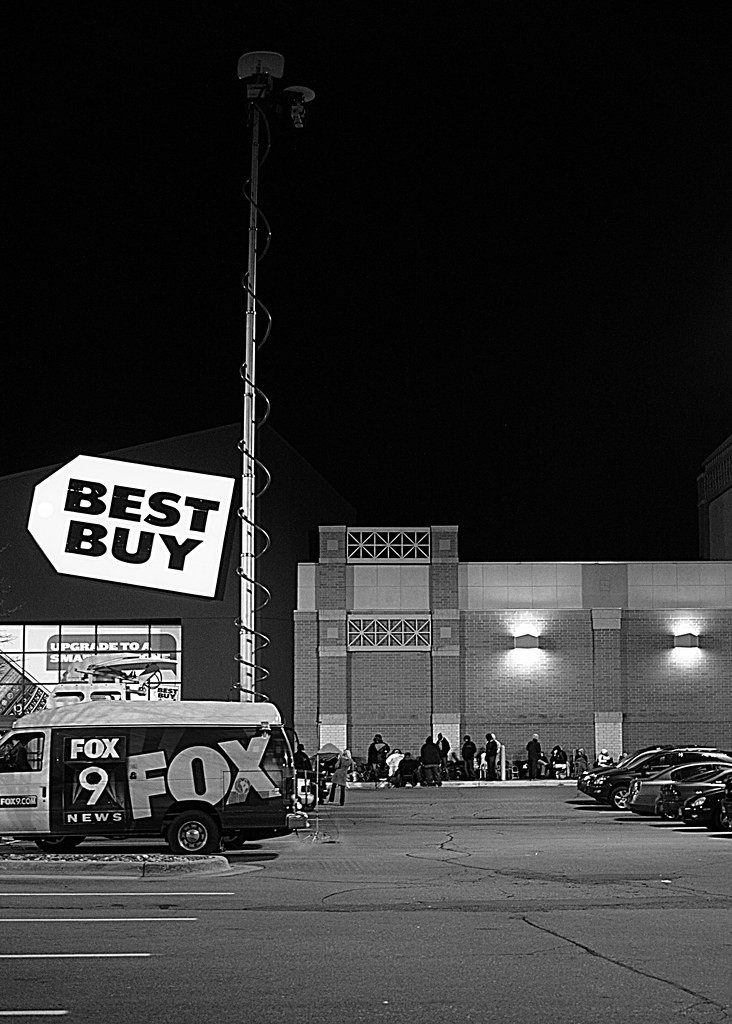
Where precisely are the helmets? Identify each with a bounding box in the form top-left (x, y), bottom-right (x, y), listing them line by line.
top-left (532, 734), bottom-right (539, 741)
top-left (578, 748), bottom-right (584, 755)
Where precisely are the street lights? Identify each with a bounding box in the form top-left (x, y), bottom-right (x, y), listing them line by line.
top-left (235, 52), bottom-right (315, 698)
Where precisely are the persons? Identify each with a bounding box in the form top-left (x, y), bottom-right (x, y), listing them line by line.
top-left (315, 734), bottom-right (422, 788)
top-left (420, 736), bottom-right (442, 787)
top-left (326, 750), bottom-right (354, 806)
top-left (525, 734), bottom-right (541, 781)
top-left (461, 736), bottom-right (478, 780)
top-left (539, 745), bottom-right (628, 779)
top-left (451, 732), bottom-right (501, 781)
top-left (293, 743), bottom-right (314, 780)
top-left (8, 745), bottom-right (32, 769)
top-left (436, 733), bottom-right (450, 778)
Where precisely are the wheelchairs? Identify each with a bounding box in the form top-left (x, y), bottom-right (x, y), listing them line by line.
top-left (396, 766), bottom-right (423, 786)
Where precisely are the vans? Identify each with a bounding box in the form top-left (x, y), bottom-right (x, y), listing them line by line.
top-left (0, 700), bottom-right (309, 856)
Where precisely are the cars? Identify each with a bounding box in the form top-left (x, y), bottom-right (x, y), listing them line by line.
top-left (578, 744), bottom-right (732, 820)
top-left (679, 780), bottom-right (732, 831)
